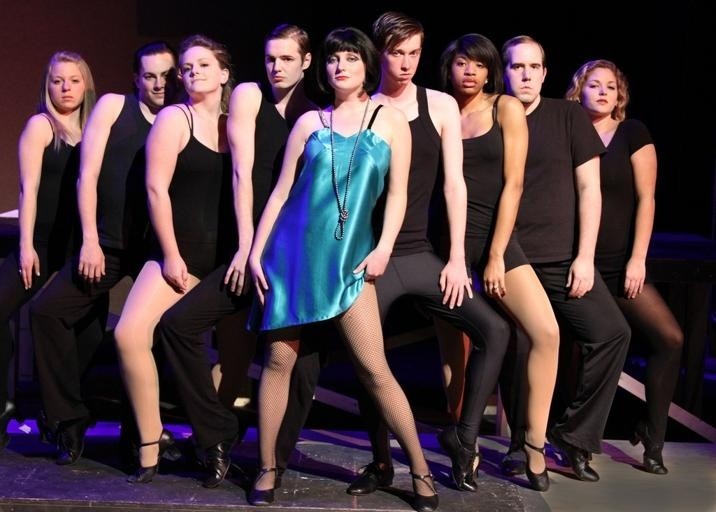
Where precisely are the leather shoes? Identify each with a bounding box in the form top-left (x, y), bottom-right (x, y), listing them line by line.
top-left (273, 458), bottom-right (288, 490)
top-left (56, 410), bottom-right (99, 467)
top-left (116, 423), bottom-right (137, 462)
top-left (346, 461), bottom-right (396, 495)
top-left (202, 421), bottom-right (243, 488)
top-left (41, 426), bottom-right (57, 446)
top-left (546, 429), bottom-right (601, 481)
top-left (518, 431), bottom-right (555, 492)
top-left (1, 429), bottom-right (11, 453)
top-left (501, 443), bottom-right (530, 476)
top-left (439, 425), bottom-right (482, 494)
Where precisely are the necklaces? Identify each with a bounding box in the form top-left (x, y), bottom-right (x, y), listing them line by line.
top-left (329, 98), bottom-right (371, 241)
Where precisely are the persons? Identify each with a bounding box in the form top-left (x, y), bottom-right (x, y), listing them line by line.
top-left (248, 28), bottom-right (439, 512)
top-left (499, 36), bottom-right (632, 483)
top-left (345, 12), bottom-right (511, 495)
top-left (431, 34), bottom-right (561, 491)
top-left (113, 34), bottom-right (233, 484)
top-left (0, 51), bottom-right (99, 451)
top-left (30, 47), bottom-right (177, 465)
top-left (566, 59), bottom-right (684, 473)
top-left (158, 27), bottom-right (326, 492)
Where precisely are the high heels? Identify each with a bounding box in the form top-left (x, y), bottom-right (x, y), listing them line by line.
top-left (127, 429), bottom-right (175, 485)
top-left (472, 440), bottom-right (481, 481)
top-left (410, 467), bottom-right (440, 511)
top-left (249, 463), bottom-right (280, 507)
top-left (629, 421), bottom-right (669, 474)
